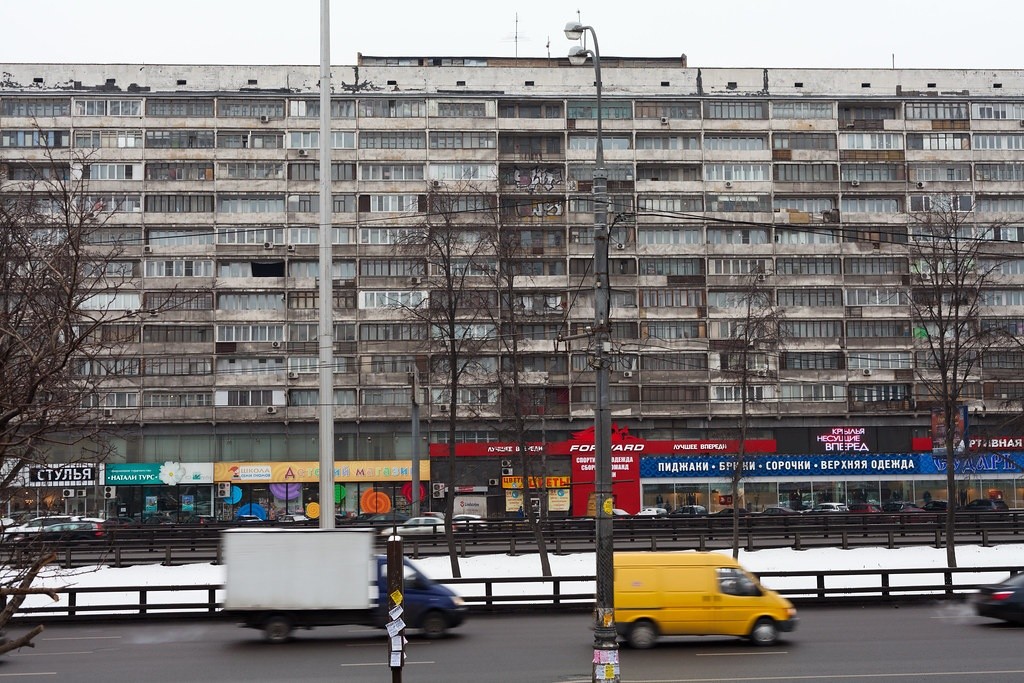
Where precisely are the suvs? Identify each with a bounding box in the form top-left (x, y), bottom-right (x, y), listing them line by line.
top-left (847, 503), bottom-right (881, 513)
top-left (800, 502), bottom-right (849, 512)
top-left (959, 498), bottom-right (1010, 512)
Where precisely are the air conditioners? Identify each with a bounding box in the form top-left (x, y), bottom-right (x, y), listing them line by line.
top-left (433, 180), bottom-right (444, 188)
top-left (757, 273), bottom-right (765, 280)
top-left (502, 459), bottom-right (512, 468)
top-left (259, 115), bottom-right (268, 123)
top-left (757, 369), bottom-right (767, 377)
top-left (623, 371), bottom-right (633, 377)
top-left (921, 272), bottom-right (932, 280)
top-left (77, 490), bottom-right (87, 497)
top-left (288, 370), bottom-right (299, 378)
top-left (264, 242), bottom-right (273, 249)
top-left (62, 489), bottom-right (74, 498)
top-left (87, 211), bottom-right (100, 221)
top-left (917, 182), bottom-right (925, 189)
top-left (143, 244), bottom-right (153, 253)
top-left (851, 179), bottom-right (860, 186)
top-left (501, 467), bottom-right (514, 475)
top-left (439, 404), bottom-right (451, 411)
top-left (103, 409), bottom-right (113, 416)
top-left (1021, 120), bottom-right (1024, 127)
top-left (432, 482), bottom-right (445, 499)
top-left (127, 309), bottom-right (137, 318)
top-left (266, 406), bottom-right (277, 414)
top-left (272, 341), bottom-right (280, 348)
top-left (411, 277), bottom-right (422, 284)
top-left (150, 308), bottom-right (158, 316)
top-left (288, 244), bottom-right (295, 252)
top-left (489, 478), bottom-right (499, 486)
top-left (298, 149), bottom-right (308, 156)
top-left (862, 369), bottom-right (872, 376)
top-left (104, 486), bottom-right (116, 500)
top-left (616, 243), bottom-right (626, 250)
top-left (919, 93), bottom-right (927, 96)
top-left (661, 116), bottom-right (668, 124)
top-left (724, 181), bottom-right (733, 187)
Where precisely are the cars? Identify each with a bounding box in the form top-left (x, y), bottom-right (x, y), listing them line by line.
top-left (334, 512), bottom-right (490, 536)
top-left (670, 506), bottom-right (708, 518)
top-left (276, 515), bottom-right (309, 521)
top-left (236, 515), bottom-right (263, 523)
top-left (635, 508), bottom-right (667, 519)
top-left (972, 572), bottom-right (1024, 625)
top-left (879, 500), bottom-right (958, 512)
top-left (581, 508), bottom-right (632, 523)
top-left (0, 513), bottom-right (220, 540)
top-left (754, 507), bottom-right (799, 516)
top-left (709, 509), bottom-right (752, 518)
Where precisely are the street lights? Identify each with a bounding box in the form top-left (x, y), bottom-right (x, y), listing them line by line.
top-left (564, 20), bottom-right (622, 682)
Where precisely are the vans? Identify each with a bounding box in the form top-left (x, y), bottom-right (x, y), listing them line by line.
top-left (611, 552), bottom-right (801, 647)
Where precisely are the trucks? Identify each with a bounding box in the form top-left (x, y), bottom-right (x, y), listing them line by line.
top-left (218, 527), bottom-right (468, 637)
